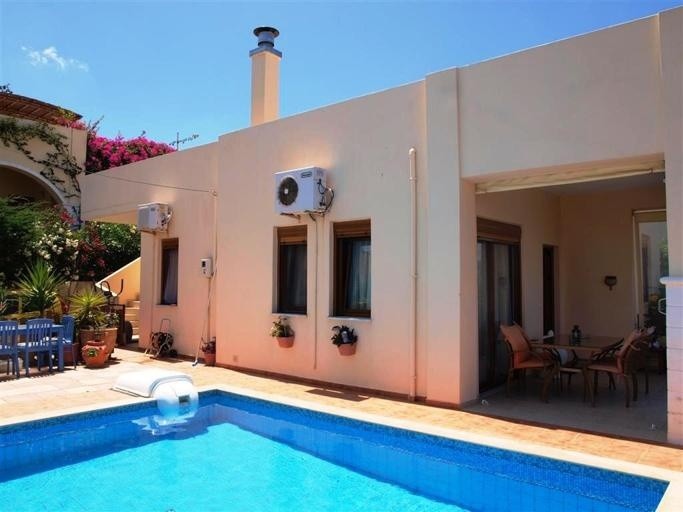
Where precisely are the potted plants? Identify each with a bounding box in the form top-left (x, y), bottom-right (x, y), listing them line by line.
top-left (197, 336), bottom-right (216, 366)
top-left (330, 324), bottom-right (358, 356)
top-left (268, 318), bottom-right (294, 348)
top-left (63, 286), bottom-right (121, 368)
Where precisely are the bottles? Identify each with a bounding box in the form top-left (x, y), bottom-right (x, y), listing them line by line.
top-left (572, 325), bottom-right (581, 339)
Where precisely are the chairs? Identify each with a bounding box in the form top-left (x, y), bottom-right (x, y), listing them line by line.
top-left (499, 321), bottom-right (655, 408)
top-left (0, 315), bottom-right (76, 379)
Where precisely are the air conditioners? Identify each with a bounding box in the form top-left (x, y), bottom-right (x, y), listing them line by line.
top-left (272, 166), bottom-right (327, 214)
top-left (135, 201), bottom-right (168, 232)
